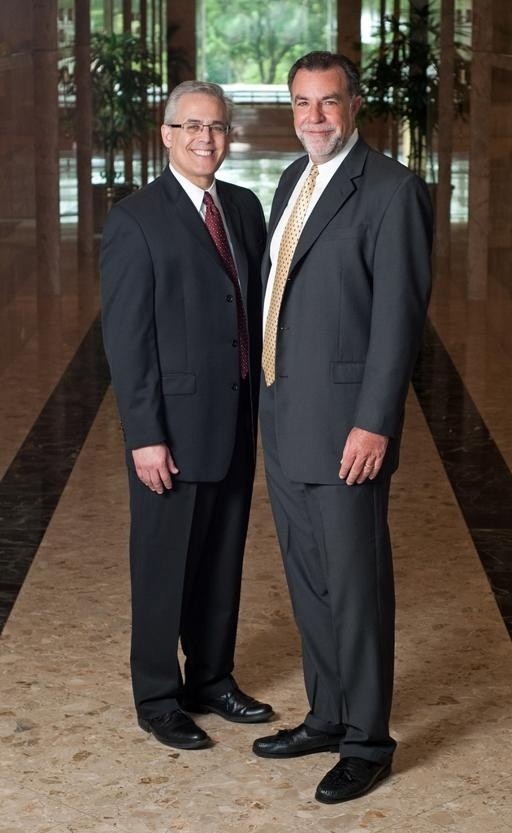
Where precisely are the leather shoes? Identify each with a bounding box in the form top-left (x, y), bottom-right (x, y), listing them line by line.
top-left (315, 757), bottom-right (391, 803)
top-left (252, 724), bottom-right (339, 759)
top-left (180, 686), bottom-right (274, 722)
top-left (138, 710), bottom-right (211, 750)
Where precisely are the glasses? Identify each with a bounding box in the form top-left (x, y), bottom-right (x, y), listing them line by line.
top-left (167, 121), bottom-right (231, 136)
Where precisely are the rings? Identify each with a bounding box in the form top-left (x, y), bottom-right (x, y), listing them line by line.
top-left (364, 463), bottom-right (374, 469)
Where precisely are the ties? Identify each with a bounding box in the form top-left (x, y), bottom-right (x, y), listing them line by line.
top-left (261, 164), bottom-right (319, 387)
top-left (202, 192), bottom-right (249, 380)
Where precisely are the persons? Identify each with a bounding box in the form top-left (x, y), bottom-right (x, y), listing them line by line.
top-left (98, 79), bottom-right (272, 751)
top-left (252, 53), bottom-right (433, 805)
